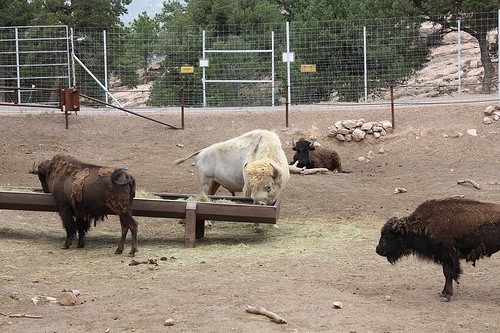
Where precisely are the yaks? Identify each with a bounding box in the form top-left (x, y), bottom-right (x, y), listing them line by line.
top-left (173, 130), bottom-right (290, 233)
top-left (28, 154), bottom-right (139, 257)
top-left (289, 138), bottom-right (353, 173)
top-left (376, 199), bottom-right (500, 302)
